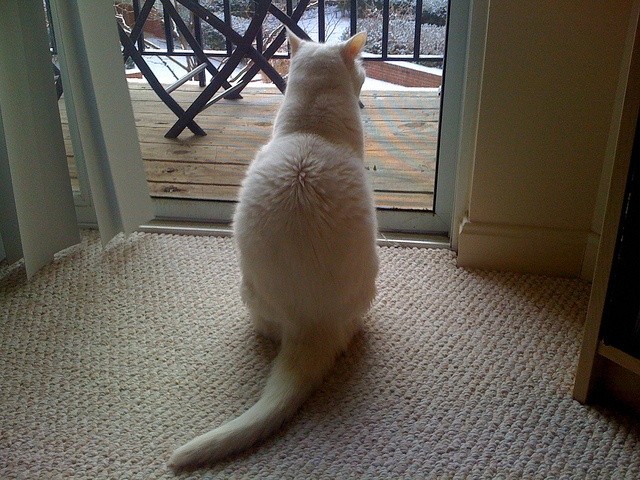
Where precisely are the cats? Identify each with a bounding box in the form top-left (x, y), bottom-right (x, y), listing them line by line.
top-left (165, 24), bottom-right (380, 469)
